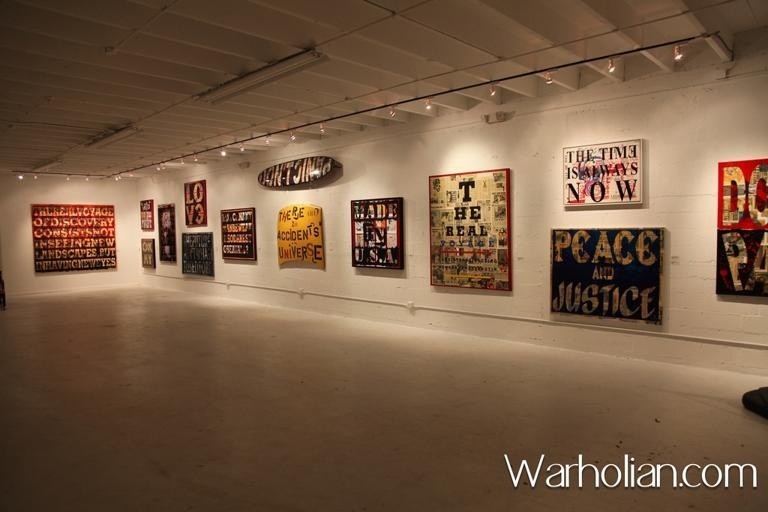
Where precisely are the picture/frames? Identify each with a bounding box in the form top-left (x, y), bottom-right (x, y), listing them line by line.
top-left (549, 225), bottom-right (666, 329)
top-left (557, 136), bottom-right (647, 209)
top-left (140, 237), bottom-right (156, 270)
top-left (157, 201), bottom-right (177, 262)
top-left (350, 197), bottom-right (404, 271)
top-left (182, 179), bottom-right (210, 228)
top-left (29, 201), bottom-right (119, 273)
top-left (426, 167), bottom-right (515, 293)
top-left (181, 231), bottom-right (216, 277)
top-left (139, 197), bottom-right (155, 231)
top-left (220, 206), bottom-right (257, 261)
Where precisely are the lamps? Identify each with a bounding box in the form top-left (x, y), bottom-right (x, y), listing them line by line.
top-left (15, 42), bottom-right (687, 185)
